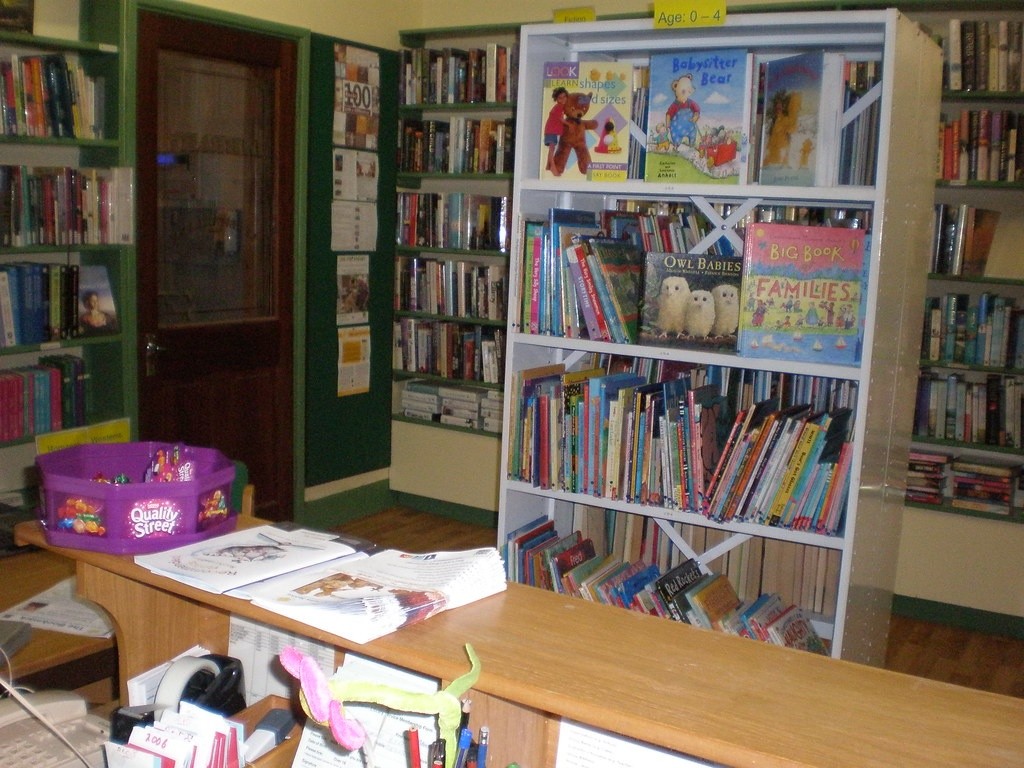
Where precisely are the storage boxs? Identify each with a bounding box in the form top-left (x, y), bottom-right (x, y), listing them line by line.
top-left (34, 441), bottom-right (239, 555)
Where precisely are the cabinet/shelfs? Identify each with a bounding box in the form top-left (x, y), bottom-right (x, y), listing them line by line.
top-left (0, 0), bottom-right (138, 557)
top-left (497, 7), bottom-right (943, 668)
top-left (392, 0), bottom-right (1024, 524)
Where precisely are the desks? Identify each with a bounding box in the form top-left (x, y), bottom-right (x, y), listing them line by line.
top-left (15, 512), bottom-right (1024, 768)
top-left (0, 551), bottom-right (117, 682)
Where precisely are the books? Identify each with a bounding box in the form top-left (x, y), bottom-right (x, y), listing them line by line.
top-left (389, 46), bottom-right (518, 429)
top-left (903, 13), bottom-right (1024, 519)
top-left (503, 33), bottom-right (884, 661)
top-left (0, 50), bottom-right (129, 449)
top-left (134, 524), bottom-right (508, 647)
